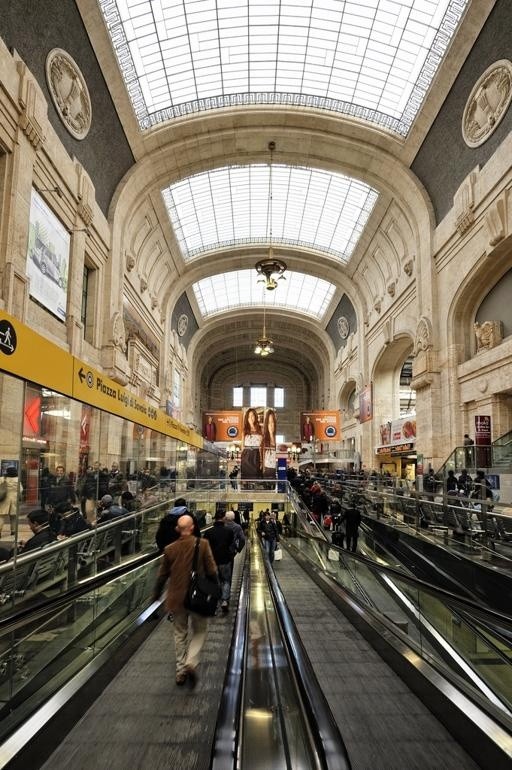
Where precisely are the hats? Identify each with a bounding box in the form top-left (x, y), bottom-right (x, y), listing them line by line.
top-left (212, 510), bottom-right (226, 520)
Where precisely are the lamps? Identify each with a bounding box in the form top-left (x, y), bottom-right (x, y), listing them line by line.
top-left (256, 142), bottom-right (289, 292)
top-left (252, 284), bottom-right (274, 357)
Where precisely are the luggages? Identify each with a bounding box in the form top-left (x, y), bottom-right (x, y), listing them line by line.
top-left (332, 525), bottom-right (343, 548)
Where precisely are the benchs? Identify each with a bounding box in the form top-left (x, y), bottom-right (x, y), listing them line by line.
top-left (1, 500), bottom-right (159, 640)
top-left (387, 489), bottom-right (512, 558)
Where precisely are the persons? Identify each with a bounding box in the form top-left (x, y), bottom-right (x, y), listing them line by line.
top-left (256, 509), bottom-right (283, 564)
top-left (263, 409), bottom-right (277, 478)
top-left (1, 461), bottom-right (176, 564)
top-left (338, 504), bottom-right (361, 553)
top-left (241, 408), bottom-right (263, 478)
top-left (204, 417), bottom-right (217, 442)
top-left (154, 498), bottom-right (250, 613)
top-left (341, 466), bottom-right (393, 519)
top-left (425, 468), bottom-right (492, 518)
top-left (152, 515), bottom-right (218, 683)
top-left (303, 416), bottom-right (315, 444)
top-left (463, 434), bottom-right (474, 468)
top-left (286, 465), bottom-right (341, 531)
top-left (228, 466), bottom-right (238, 489)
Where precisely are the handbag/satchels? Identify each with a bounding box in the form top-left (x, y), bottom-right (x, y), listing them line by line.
top-left (274, 547), bottom-right (283, 560)
top-left (185, 536), bottom-right (221, 618)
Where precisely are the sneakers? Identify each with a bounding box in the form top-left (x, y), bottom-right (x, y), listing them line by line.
top-left (221, 601), bottom-right (229, 615)
